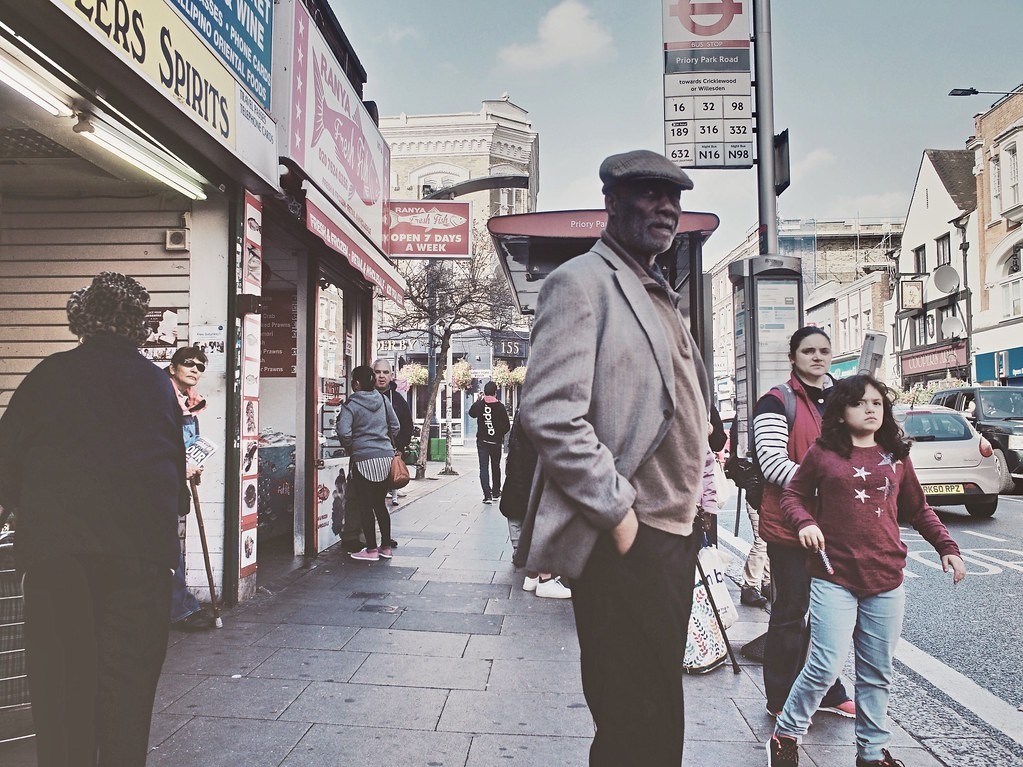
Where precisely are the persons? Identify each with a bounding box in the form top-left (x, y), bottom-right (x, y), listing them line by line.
top-left (499, 401), bottom-right (573, 598)
top-left (336, 366), bottom-right (400, 560)
top-left (766, 372), bottom-right (967, 767)
top-left (754, 324), bottom-right (857, 723)
top-left (0, 271), bottom-right (191, 767)
top-left (342, 358), bottom-right (414, 551)
top-left (160, 346), bottom-right (215, 631)
top-left (519, 150), bottom-right (714, 766)
top-left (698, 395), bottom-right (770, 606)
top-left (970, 397), bottom-right (995, 418)
top-left (468, 381), bottom-right (511, 504)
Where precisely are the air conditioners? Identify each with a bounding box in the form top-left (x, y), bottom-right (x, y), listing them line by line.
top-left (163, 228), bottom-right (190, 252)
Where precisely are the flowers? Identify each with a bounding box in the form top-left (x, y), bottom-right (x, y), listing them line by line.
top-left (441, 357), bottom-right (478, 392)
top-left (395, 361), bottom-right (428, 395)
top-left (482, 359), bottom-right (527, 390)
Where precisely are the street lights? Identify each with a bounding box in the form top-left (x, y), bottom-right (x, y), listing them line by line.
top-left (423, 173), bottom-right (529, 460)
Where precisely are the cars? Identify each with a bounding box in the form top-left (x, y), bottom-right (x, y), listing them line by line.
top-left (891, 404), bottom-right (999, 517)
top-left (720, 412), bottom-right (735, 430)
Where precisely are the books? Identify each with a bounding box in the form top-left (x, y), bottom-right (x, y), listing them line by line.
top-left (186, 435), bottom-right (221, 479)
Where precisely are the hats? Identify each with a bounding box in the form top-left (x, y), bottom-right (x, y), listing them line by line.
top-left (599, 149), bottom-right (693, 195)
top-left (67, 269), bottom-right (153, 342)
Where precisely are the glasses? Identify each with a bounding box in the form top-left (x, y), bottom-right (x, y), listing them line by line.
top-left (179, 359), bottom-right (206, 372)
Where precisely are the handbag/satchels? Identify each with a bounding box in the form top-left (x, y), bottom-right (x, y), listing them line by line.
top-left (695, 530), bottom-right (738, 630)
top-left (683, 581), bottom-right (728, 673)
top-left (728, 460), bottom-right (754, 489)
top-left (390, 454), bottom-right (410, 489)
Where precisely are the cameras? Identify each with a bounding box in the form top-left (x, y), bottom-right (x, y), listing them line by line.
top-left (724, 453), bottom-right (763, 509)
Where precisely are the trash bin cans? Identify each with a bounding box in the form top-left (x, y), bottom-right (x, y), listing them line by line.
top-left (430, 438), bottom-right (446, 462)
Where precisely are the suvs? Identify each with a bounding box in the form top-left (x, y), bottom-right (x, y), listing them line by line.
top-left (930, 387), bottom-right (1023, 496)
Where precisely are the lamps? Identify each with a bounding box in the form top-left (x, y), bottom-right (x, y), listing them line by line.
top-left (71, 113), bottom-right (95, 136)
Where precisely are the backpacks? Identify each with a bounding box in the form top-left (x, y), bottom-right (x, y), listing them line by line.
top-left (745, 384), bottom-right (797, 510)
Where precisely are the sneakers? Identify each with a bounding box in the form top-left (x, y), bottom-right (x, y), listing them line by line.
top-left (766, 732), bottom-right (799, 767)
top-left (766, 703), bottom-right (785, 722)
top-left (741, 584), bottom-right (767, 606)
top-left (535, 576), bottom-right (571, 599)
top-left (761, 579), bottom-right (772, 599)
top-left (817, 698), bottom-right (856, 719)
top-left (855, 748), bottom-right (905, 767)
top-left (376, 544), bottom-right (393, 559)
top-left (194, 609), bottom-right (223, 628)
top-left (523, 575), bottom-right (538, 591)
top-left (169, 613), bottom-right (210, 632)
top-left (351, 547), bottom-right (379, 561)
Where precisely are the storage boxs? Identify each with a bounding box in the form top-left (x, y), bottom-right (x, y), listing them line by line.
top-left (401, 450), bottom-right (417, 464)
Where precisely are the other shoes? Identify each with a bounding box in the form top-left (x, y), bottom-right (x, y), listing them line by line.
top-left (390, 539), bottom-right (398, 547)
top-left (482, 498), bottom-right (492, 504)
top-left (492, 490), bottom-right (501, 500)
top-left (341, 542), bottom-right (367, 553)
